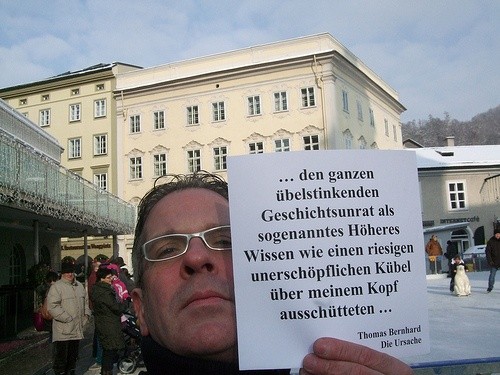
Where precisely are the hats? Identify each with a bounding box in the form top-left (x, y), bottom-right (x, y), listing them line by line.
top-left (58, 262), bottom-right (76, 273)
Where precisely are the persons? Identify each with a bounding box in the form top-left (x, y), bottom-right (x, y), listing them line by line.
top-left (449, 256), bottom-right (470, 291)
top-left (485, 229), bottom-right (500, 293)
top-left (426, 235), bottom-right (444, 274)
top-left (90, 268), bottom-right (131, 375)
top-left (87, 261), bottom-right (102, 358)
top-left (444, 240), bottom-right (458, 272)
top-left (100, 256), bottom-right (130, 305)
top-left (46, 262), bottom-right (92, 374)
top-left (113, 168), bottom-right (413, 375)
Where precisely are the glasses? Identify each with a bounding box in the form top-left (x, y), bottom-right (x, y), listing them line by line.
top-left (142, 225), bottom-right (231, 261)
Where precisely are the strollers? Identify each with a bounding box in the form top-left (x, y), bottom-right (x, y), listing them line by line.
top-left (119, 313), bottom-right (142, 374)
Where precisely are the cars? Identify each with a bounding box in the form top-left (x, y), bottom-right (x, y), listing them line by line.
top-left (463, 244), bottom-right (487, 260)
